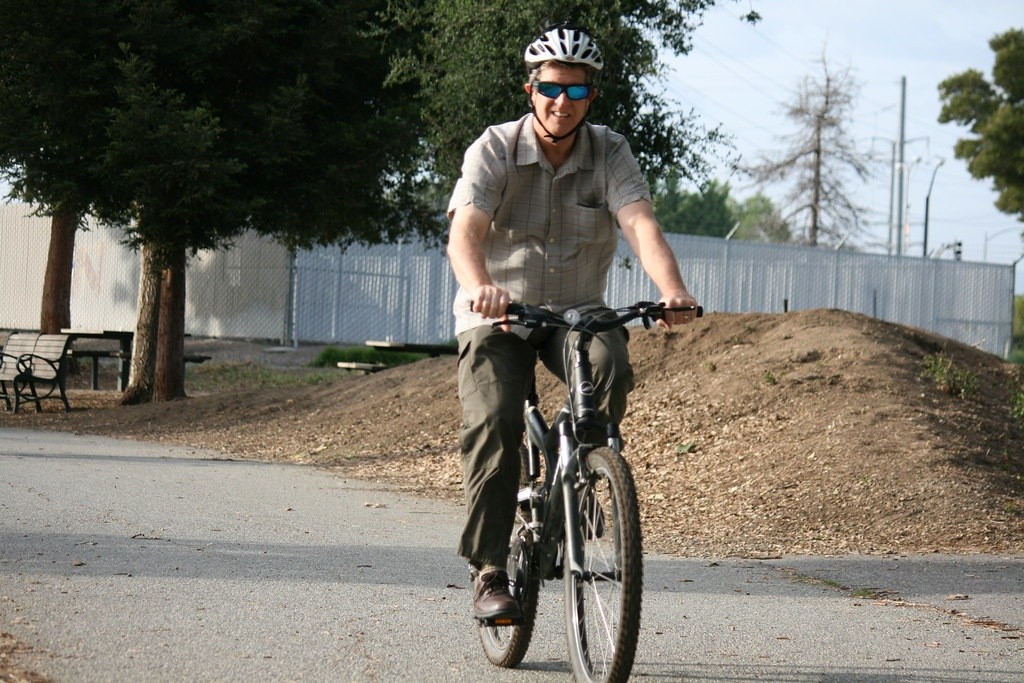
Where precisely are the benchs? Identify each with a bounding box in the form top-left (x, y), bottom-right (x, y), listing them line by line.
top-left (0, 329), bottom-right (78, 414)
top-left (336, 362), bottom-right (386, 372)
top-left (71, 350), bottom-right (213, 365)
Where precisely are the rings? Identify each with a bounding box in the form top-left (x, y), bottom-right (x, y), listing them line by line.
top-left (683, 316), bottom-right (691, 319)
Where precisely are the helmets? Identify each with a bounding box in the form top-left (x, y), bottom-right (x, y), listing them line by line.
top-left (524, 26), bottom-right (604, 77)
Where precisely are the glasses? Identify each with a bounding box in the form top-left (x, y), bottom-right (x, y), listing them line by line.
top-left (532, 81), bottom-right (592, 100)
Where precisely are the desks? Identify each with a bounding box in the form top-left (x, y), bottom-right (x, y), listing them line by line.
top-left (60, 328), bottom-right (191, 392)
top-left (366, 341), bottom-right (459, 358)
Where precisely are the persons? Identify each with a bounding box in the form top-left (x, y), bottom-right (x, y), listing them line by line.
top-left (446, 29), bottom-right (697, 620)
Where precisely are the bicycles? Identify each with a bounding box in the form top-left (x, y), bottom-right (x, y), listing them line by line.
top-left (476, 299), bottom-right (706, 683)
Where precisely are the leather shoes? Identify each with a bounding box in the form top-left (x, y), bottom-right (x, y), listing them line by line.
top-left (582, 492), bottom-right (604, 539)
top-left (473, 569), bottom-right (522, 620)
